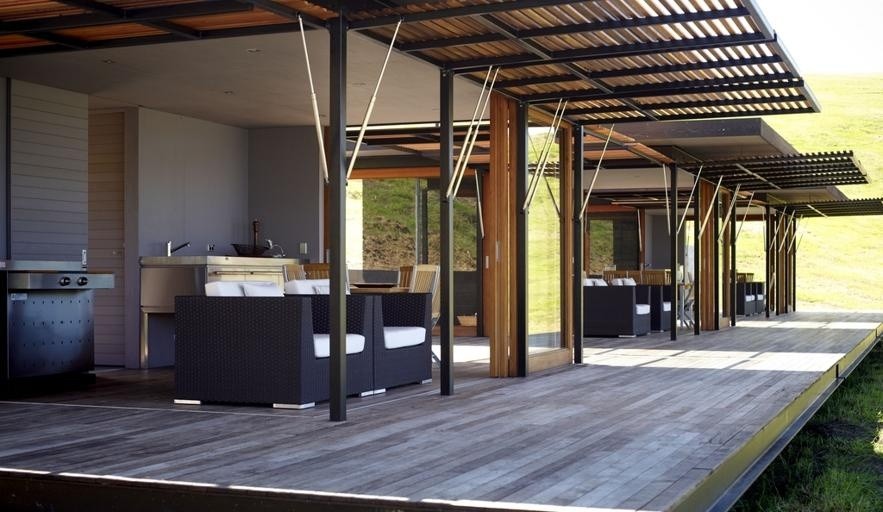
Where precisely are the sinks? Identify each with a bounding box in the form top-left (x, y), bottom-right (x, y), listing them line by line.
top-left (231, 243), bottom-right (268, 257)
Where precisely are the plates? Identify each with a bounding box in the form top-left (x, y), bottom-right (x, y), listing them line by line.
top-left (349, 282), bottom-right (398, 288)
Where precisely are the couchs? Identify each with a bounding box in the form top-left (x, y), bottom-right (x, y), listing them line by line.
top-left (174, 279), bottom-right (433, 408)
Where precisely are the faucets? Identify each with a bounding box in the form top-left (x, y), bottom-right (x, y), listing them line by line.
top-left (640, 263), bottom-right (651, 270)
top-left (266, 238), bottom-right (273, 248)
top-left (166, 240), bottom-right (191, 256)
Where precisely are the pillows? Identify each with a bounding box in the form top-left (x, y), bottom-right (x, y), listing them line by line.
top-left (313, 284), bottom-right (352, 295)
top-left (240, 282), bottom-right (283, 296)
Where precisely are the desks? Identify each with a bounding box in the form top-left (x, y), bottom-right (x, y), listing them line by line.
top-left (137, 253), bottom-right (301, 373)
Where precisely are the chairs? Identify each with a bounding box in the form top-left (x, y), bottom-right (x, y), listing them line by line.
top-left (581, 269), bottom-right (687, 338)
top-left (283, 262), bottom-right (441, 366)
top-left (735, 272), bottom-right (765, 317)
